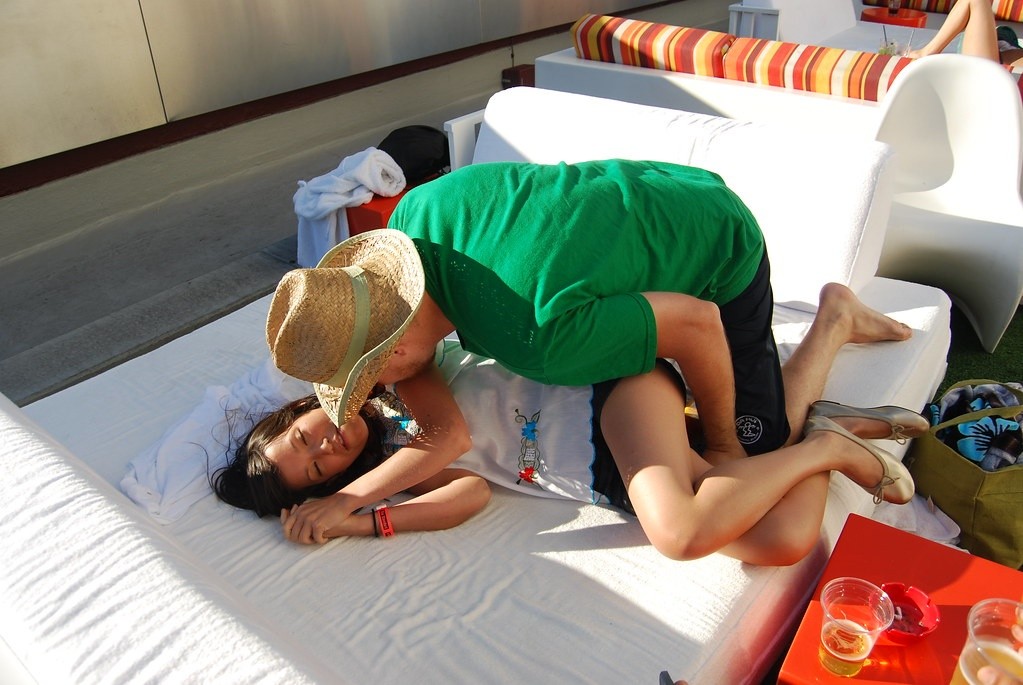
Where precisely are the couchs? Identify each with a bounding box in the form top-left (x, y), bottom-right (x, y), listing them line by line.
top-left (862, 0), bottom-right (1023, 38)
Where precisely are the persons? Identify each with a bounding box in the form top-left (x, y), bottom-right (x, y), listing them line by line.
top-left (210, 339), bottom-right (930, 562)
top-left (266, 159), bottom-right (913, 465)
top-left (898, 0), bottom-right (1023, 64)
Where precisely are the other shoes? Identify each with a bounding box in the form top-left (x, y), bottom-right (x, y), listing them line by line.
top-left (804, 416), bottom-right (915, 505)
top-left (806, 399), bottom-right (929, 440)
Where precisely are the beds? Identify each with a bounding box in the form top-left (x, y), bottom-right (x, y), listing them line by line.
top-left (0, 88), bottom-right (951, 685)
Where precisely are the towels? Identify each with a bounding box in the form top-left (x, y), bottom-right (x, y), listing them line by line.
top-left (293, 145), bottom-right (406, 269)
top-left (118, 355), bottom-right (293, 527)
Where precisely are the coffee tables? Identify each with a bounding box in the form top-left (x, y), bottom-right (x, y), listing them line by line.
top-left (817, 21), bottom-right (964, 58)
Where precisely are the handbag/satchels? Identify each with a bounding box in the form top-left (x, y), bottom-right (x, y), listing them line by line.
top-left (907, 375), bottom-right (1023, 570)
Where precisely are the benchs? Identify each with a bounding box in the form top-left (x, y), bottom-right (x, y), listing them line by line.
top-left (534, 14), bottom-right (1023, 135)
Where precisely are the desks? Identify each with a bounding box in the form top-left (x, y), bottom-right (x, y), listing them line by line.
top-left (861, 8), bottom-right (927, 28)
top-left (776, 513), bottom-right (1023, 685)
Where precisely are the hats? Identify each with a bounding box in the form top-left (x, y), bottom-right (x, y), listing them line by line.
top-left (267, 228), bottom-right (426, 429)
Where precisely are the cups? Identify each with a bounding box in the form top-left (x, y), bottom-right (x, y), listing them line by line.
top-left (948, 598), bottom-right (1023, 685)
top-left (819, 577), bottom-right (894, 677)
top-left (888, 0), bottom-right (901, 16)
top-left (879, 38), bottom-right (898, 54)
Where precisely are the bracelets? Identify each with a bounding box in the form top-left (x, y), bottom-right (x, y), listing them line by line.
top-left (371, 509), bottom-right (379, 537)
top-left (376, 504), bottom-right (395, 537)
